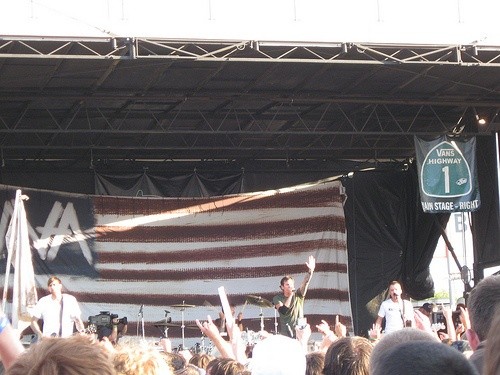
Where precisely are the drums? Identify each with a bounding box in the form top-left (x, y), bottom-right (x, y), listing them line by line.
top-left (220, 333), bottom-right (250, 358)
top-left (195, 342), bottom-right (213, 355)
top-left (176, 349), bottom-right (196, 363)
top-left (248, 333), bottom-right (273, 351)
top-left (155, 347), bottom-right (165, 353)
top-left (243, 329), bottom-right (255, 346)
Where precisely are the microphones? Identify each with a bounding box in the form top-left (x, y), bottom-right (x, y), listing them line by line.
top-left (139, 304), bottom-right (144, 316)
top-left (393, 292), bottom-right (399, 296)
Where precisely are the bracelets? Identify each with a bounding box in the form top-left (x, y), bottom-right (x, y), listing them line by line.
top-left (369, 337), bottom-right (377, 340)
top-left (238, 320), bottom-right (242, 324)
top-left (0, 316), bottom-right (9, 333)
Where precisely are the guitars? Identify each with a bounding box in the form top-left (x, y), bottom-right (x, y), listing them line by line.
top-left (29, 324), bottom-right (97, 349)
top-left (403, 319), bottom-right (412, 328)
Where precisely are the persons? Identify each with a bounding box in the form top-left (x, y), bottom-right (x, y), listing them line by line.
top-left (0, 273), bottom-right (500, 375)
top-left (272, 255), bottom-right (315, 339)
top-left (30, 276), bottom-right (85, 343)
top-left (372, 281), bottom-right (416, 332)
top-left (213, 303), bottom-right (243, 332)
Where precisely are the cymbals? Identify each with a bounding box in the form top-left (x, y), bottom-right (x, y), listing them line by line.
top-left (171, 304), bottom-right (195, 308)
top-left (251, 317), bottom-right (271, 322)
top-left (154, 322), bottom-right (182, 327)
top-left (187, 324), bottom-right (204, 329)
top-left (246, 295), bottom-right (274, 308)
